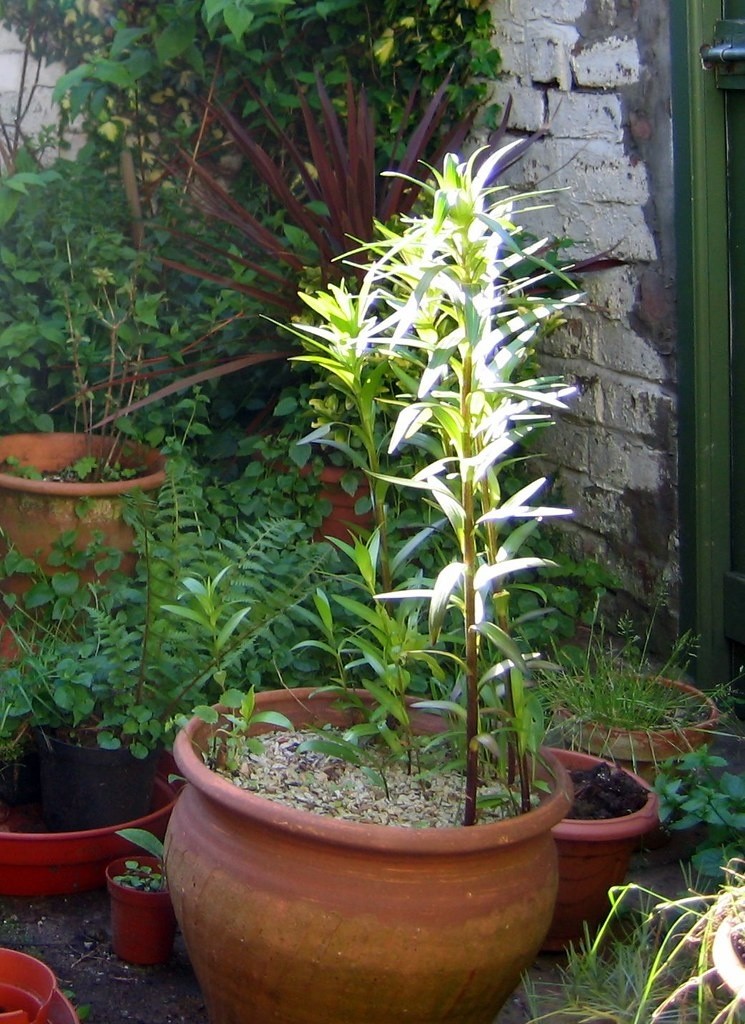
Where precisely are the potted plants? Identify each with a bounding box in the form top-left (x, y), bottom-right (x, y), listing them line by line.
top-left (0, 0), bottom-right (745, 1024)
